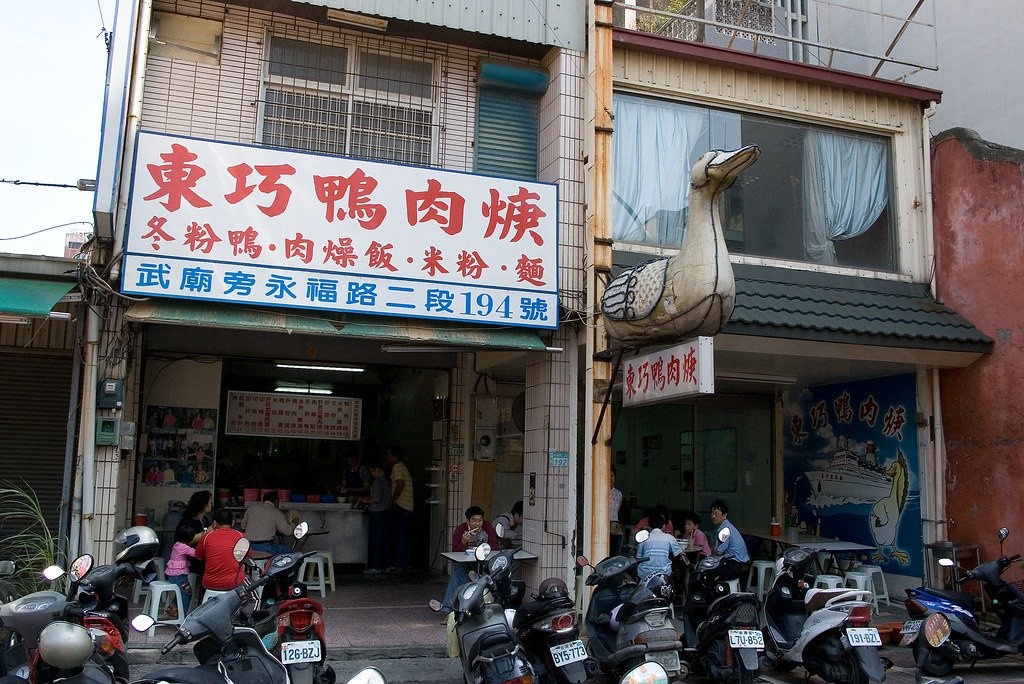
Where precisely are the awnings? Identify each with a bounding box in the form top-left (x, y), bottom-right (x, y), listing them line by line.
top-left (0, 277), bottom-right (78, 318)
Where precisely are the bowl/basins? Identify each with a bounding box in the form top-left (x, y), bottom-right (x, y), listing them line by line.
top-left (337, 497), bottom-right (346, 503)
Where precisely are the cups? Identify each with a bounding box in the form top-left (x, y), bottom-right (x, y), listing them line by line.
top-left (770, 522), bottom-right (780, 536)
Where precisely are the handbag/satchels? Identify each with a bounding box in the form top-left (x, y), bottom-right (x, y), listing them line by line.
top-left (611, 522), bottom-right (623, 535)
top-left (615, 587), bottom-right (656, 622)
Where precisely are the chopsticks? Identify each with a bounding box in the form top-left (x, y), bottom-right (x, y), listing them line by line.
top-left (468, 528), bottom-right (479, 534)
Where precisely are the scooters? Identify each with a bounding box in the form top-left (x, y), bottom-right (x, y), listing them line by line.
top-left (898, 527), bottom-right (1024, 678)
top-left (0, 520), bottom-right (389, 684)
top-left (429, 523), bottom-right (895, 684)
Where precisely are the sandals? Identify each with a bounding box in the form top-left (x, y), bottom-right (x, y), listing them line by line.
top-left (165, 606), bottom-right (179, 617)
top-left (441, 616), bottom-right (449, 624)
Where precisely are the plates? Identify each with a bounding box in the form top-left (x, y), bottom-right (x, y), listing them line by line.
top-left (466, 549), bottom-right (475, 555)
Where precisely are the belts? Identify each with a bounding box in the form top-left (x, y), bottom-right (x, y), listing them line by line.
top-left (248, 540), bottom-right (271, 544)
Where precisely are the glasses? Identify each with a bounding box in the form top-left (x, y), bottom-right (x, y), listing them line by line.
top-left (711, 511), bottom-right (724, 517)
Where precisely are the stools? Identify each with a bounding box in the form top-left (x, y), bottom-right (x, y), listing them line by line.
top-left (746, 560), bottom-right (775, 602)
top-left (202, 589), bottom-right (229, 604)
top-left (297, 556), bottom-right (326, 597)
top-left (164, 573), bottom-right (200, 615)
top-left (307, 551), bottom-right (335, 591)
top-left (845, 572), bottom-right (880, 616)
top-left (248, 559), bottom-right (268, 609)
top-left (143, 580), bottom-right (184, 637)
top-left (131, 557), bottom-right (167, 602)
top-left (860, 565), bottom-right (890, 606)
top-left (716, 578), bottom-right (742, 595)
top-left (813, 575), bottom-right (845, 589)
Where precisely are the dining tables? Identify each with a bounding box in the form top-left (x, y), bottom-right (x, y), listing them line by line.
top-left (627, 542), bottom-right (703, 593)
top-left (440, 548), bottom-right (538, 579)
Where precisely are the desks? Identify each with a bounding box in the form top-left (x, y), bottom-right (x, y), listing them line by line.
top-left (153, 525), bottom-right (177, 557)
top-left (277, 527), bottom-right (331, 551)
top-left (791, 542), bottom-right (878, 588)
top-left (740, 528), bottom-right (843, 582)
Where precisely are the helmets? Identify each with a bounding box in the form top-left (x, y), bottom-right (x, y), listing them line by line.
top-left (530, 578), bottom-right (569, 599)
top-left (113, 526), bottom-right (159, 564)
top-left (640, 572), bottom-right (676, 604)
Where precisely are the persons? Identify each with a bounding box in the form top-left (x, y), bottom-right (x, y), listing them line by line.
top-left (241, 491), bottom-right (299, 554)
top-left (706, 499), bottom-right (749, 582)
top-left (491, 501), bottom-right (523, 580)
top-left (439, 506), bottom-right (499, 625)
top-left (629, 504), bottom-right (711, 588)
top-left (145, 435), bottom-right (213, 485)
top-left (336, 444), bottom-right (414, 573)
top-left (148, 408), bottom-right (176, 428)
top-left (165, 490), bottom-right (259, 624)
top-left (609, 464), bottom-right (622, 555)
top-left (231, 454), bottom-right (265, 498)
top-left (192, 412), bottom-right (215, 430)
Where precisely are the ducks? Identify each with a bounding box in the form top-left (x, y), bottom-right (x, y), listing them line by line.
top-left (601, 144), bottom-right (762, 344)
